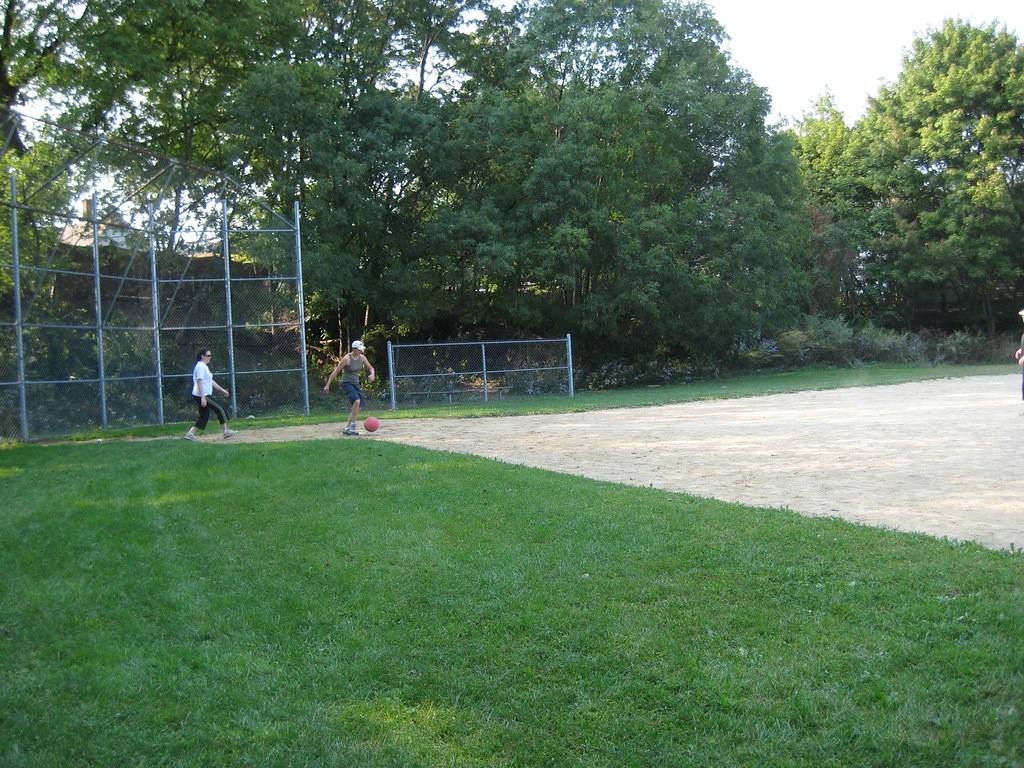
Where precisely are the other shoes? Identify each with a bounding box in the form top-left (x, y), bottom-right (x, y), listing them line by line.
top-left (343, 428), bottom-right (360, 435)
top-left (185, 433), bottom-right (200, 442)
top-left (224, 429), bottom-right (238, 438)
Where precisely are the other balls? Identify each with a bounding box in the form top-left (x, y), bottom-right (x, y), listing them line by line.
top-left (363, 417), bottom-right (379, 431)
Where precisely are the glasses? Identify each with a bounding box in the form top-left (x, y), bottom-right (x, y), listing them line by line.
top-left (204, 354), bottom-right (212, 357)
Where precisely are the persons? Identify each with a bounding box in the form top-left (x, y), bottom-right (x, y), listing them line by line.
top-left (184, 349), bottom-right (237, 442)
top-left (323, 340), bottom-right (374, 436)
top-left (1016, 311), bottom-right (1024, 416)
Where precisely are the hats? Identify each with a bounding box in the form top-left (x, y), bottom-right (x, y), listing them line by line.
top-left (352, 340), bottom-right (365, 351)
